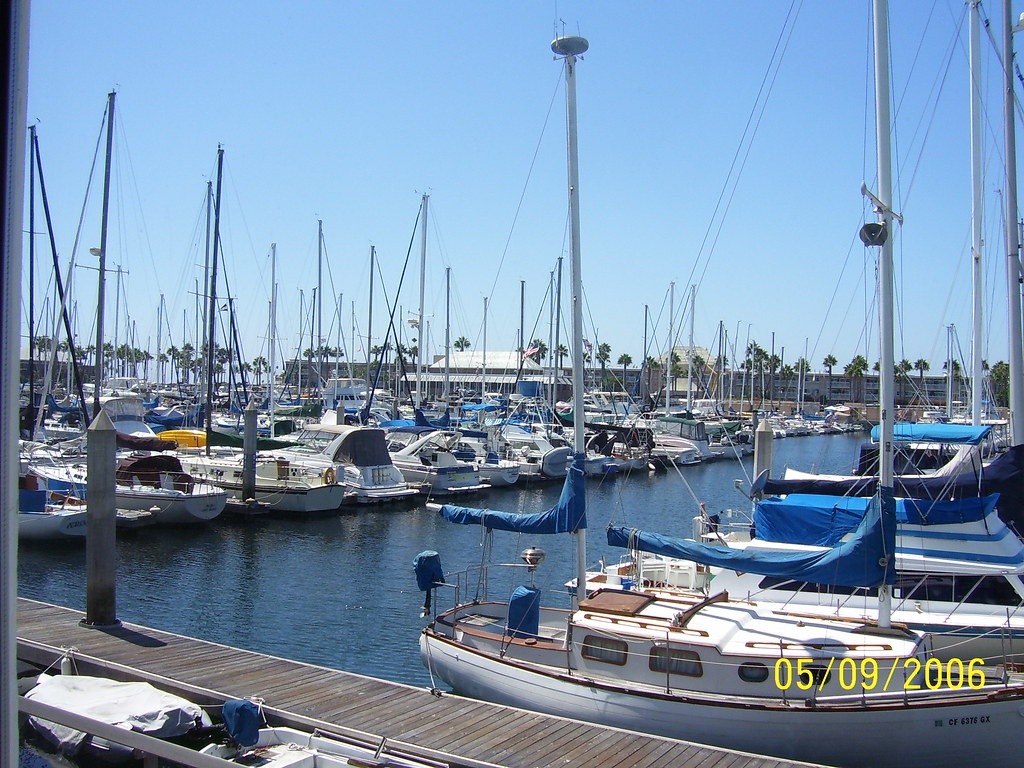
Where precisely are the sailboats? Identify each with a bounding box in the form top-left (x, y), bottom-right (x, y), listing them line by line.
top-left (20, 1), bottom-right (1024, 768)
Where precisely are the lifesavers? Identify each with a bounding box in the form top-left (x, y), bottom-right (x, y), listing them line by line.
top-left (322, 467), bottom-right (334, 484)
